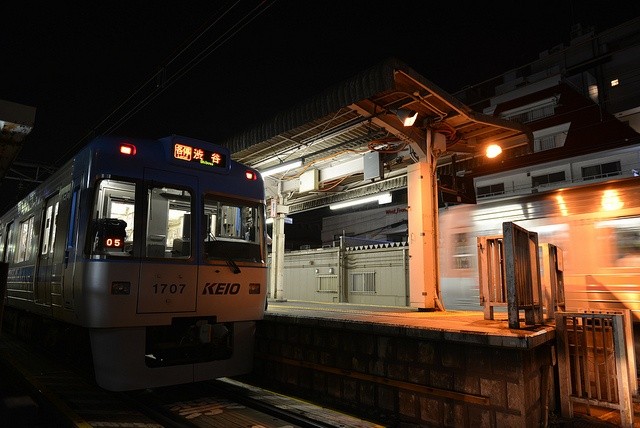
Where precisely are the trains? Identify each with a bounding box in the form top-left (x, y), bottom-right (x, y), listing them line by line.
top-left (422, 167), bottom-right (635, 317)
top-left (0, 134), bottom-right (267, 393)
